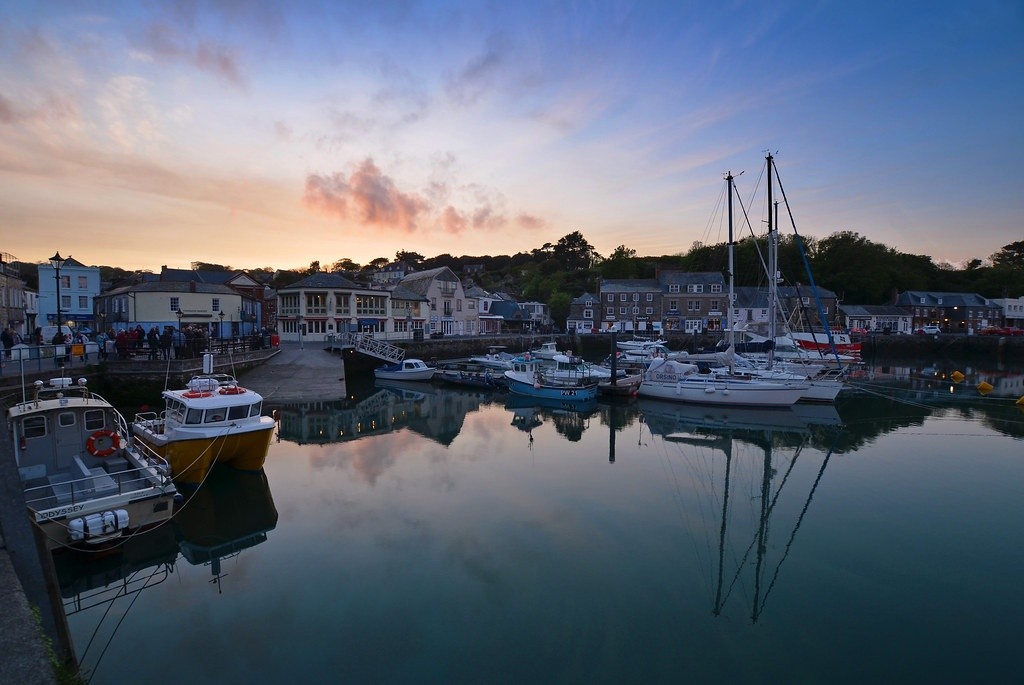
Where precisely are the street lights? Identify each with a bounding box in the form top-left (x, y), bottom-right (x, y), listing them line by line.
top-left (175, 308), bottom-right (184, 354)
top-left (49, 251), bottom-right (65, 333)
top-left (218, 309), bottom-right (225, 353)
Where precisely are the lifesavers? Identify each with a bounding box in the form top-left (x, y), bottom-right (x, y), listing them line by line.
top-left (183, 390), bottom-right (211, 398)
top-left (86, 430), bottom-right (120, 457)
top-left (219, 387), bottom-right (247, 394)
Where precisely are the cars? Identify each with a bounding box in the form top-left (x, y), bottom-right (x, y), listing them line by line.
top-left (920, 367), bottom-right (939, 377)
top-left (976, 326), bottom-right (1024, 336)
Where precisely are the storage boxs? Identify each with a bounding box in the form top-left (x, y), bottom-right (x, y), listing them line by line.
top-left (104, 457), bottom-right (129, 473)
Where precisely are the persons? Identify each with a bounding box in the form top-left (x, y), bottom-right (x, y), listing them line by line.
top-left (0, 327), bottom-right (15, 359)
top-left (249, 325), bottom-right (271, 351)
top-left (230, 329), bottom-right (239, 337)
top-left (96, 324), bottom-right (145, 362)
top-left (52, 331), bottom-right (89, 368)
top-left (147, 323), bottom-right (216, 360)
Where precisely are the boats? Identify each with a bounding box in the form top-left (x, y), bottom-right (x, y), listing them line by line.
top-left (506, 391), bottom-right (595, 451)
top-left (436, 150), bottom-right (862, 405)
top-left (177, 471), bottom-right (279, 594)
top-left (52, 520), bottom-right (177, 616)
top-left (374, 379), bottom-right (436, 401)
top-left (8, 367), bottom-right (177, 550)
top-left (132, 354), bottom-right (275, 483)
top-left (374, 359), bottom-right (436, 380)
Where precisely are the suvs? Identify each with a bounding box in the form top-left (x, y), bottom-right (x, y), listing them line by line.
top-left (914, 326), bottom-right (941, 335)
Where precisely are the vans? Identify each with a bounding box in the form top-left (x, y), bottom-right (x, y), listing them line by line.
top-left (39, 326), bottom-right (73, 344)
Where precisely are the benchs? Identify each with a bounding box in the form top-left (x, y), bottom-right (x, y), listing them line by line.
top-left (45, 454), bottom-right (120, 507)
top-left (204, 342), bottom-right (255, 354)
top-left (117, 347), bottom-right (162, 361)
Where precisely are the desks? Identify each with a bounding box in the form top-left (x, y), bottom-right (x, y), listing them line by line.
top-left (20, 463), bottom-right (47, 493)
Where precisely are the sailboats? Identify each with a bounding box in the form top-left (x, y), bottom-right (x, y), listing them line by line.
top-left (634, 399), bottom-right (844, 625)
